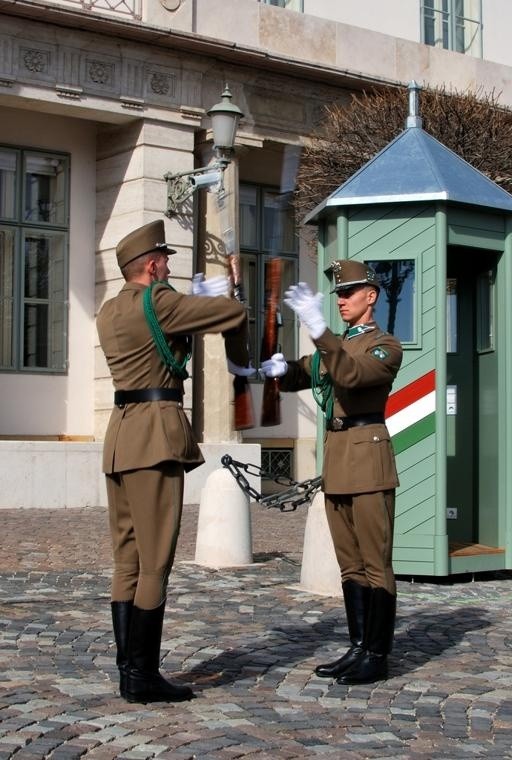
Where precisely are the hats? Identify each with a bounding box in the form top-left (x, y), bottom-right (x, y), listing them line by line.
top-left (329, 260), bottom-right (381, 296)
top-left (116, 219), bottom-right (177, 268)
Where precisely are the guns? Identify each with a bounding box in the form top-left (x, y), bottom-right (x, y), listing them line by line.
top-left (259, 255), bottom-right (281, 425)
top-left (223, 253), bottom-right (255, 431)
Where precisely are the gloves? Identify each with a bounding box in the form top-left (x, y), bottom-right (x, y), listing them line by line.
top-left (259, 352), bottom-right (290, 381)
top-left (227, 358), bottom-right (257, 377)
top-left (284, 282), bottom-right (327, 340)
top-left (190, 272), bottom-right (230, 296)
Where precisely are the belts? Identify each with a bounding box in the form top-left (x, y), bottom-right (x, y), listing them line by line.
top-left (326, 413), bottom-right (385, 433)
top-left (114, 387), bottom-right (183, 404)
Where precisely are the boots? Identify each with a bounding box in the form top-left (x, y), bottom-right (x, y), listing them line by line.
top-left (314, 579), bottom-right (395, 685)
top-left (109, 596), bottom-right (194, 703)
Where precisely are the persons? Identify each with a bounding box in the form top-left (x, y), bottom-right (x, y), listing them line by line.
top-left (255, 258), bottom-right (408, 688)
top-left (95, 216), bottom-right (249, 705)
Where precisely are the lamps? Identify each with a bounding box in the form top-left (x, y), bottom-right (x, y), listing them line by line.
top-left (163, 79), bottom-right (244, 223)
